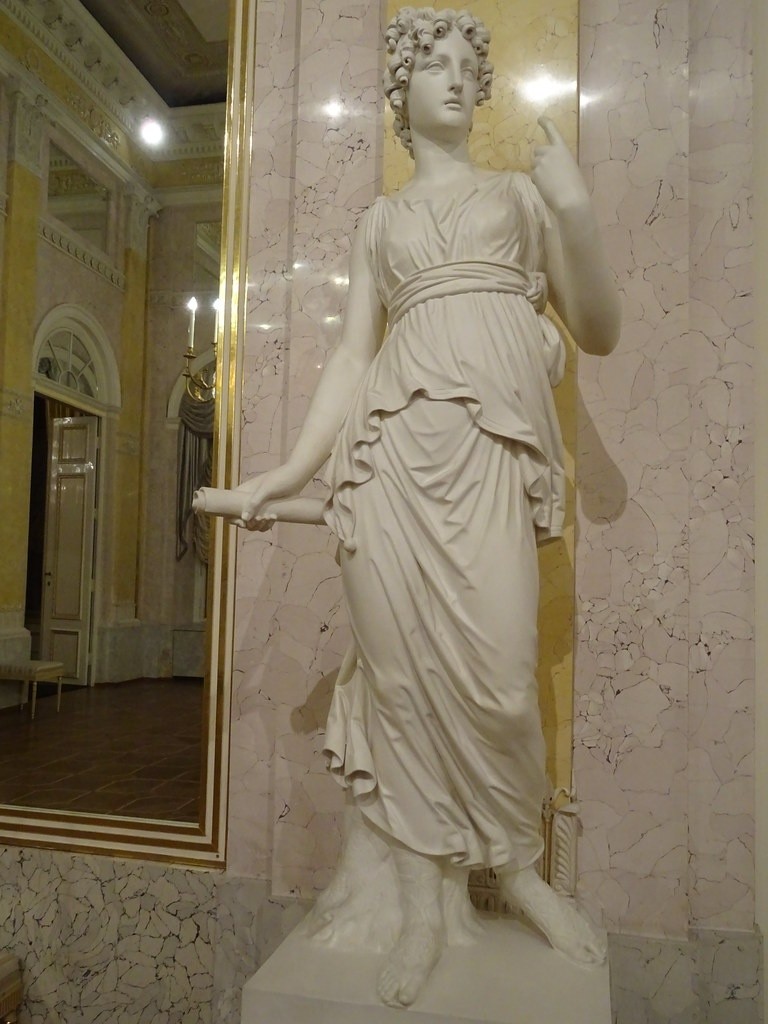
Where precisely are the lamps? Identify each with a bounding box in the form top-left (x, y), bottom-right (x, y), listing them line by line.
top-left (183, 297), bottom-right (217, 404)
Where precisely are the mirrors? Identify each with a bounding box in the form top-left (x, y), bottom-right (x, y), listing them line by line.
top-left (0, 1), bottom-right (257, 873)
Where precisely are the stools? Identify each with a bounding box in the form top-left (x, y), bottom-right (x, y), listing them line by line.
top-left (0, 660), bottom-right (66, 719)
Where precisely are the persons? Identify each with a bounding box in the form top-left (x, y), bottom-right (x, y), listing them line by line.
top-left (226, 6), bottom-right (620, 1006)
